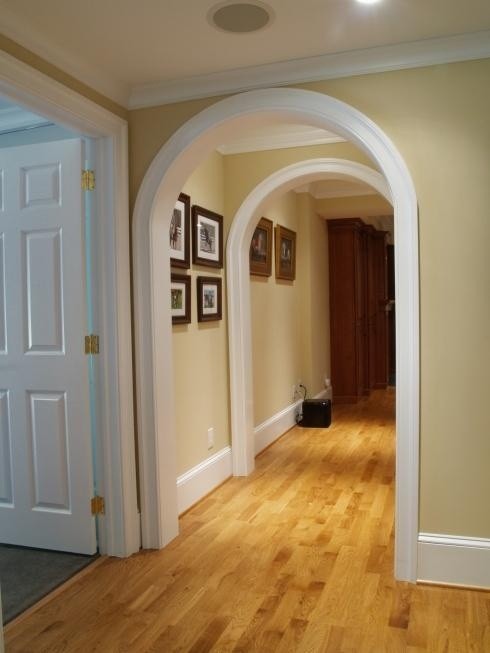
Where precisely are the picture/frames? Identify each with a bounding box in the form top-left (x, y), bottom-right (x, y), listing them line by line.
top-left (171, 192), bottom-right (224, 324)
top-left (250, 217), bottom-right (296, 282)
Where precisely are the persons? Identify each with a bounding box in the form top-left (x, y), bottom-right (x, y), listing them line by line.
top-left (169, 208), bottom-right (294, 308)
